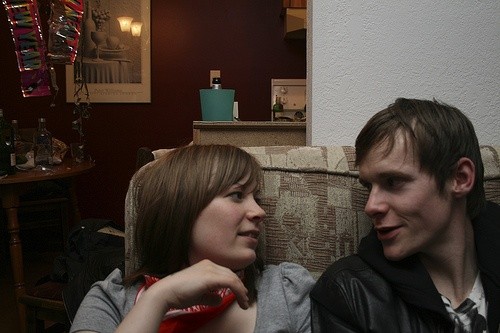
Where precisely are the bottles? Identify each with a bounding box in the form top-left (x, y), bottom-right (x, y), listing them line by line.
top-left (32, 118), bottom-right (52, 166)
top-left (25, 78), bottom-right (42, 93)
top-left (0, 108), bottom-right (16, 176)
top-left (212, 77), bottom-right (221, 89)
top-left (11, 119), bottom-right (22, 144)
top-left (274, 97), bottom-right (283, 110)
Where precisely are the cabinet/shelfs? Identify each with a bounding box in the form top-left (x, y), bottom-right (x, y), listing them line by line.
top-left (271, 79), bottom-right (307, 123)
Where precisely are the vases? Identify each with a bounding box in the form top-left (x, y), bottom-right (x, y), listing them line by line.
top-left (90, 31), bottom-right (107, 62)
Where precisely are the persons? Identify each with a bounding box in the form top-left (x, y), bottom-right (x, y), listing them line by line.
top-left (69, 143), bottom-right (318, 333)
top-left (310, 97), bottom-right (500, 333)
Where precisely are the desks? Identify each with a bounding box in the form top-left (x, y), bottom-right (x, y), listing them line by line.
top-left (193, 122), bottom-right (306, 147)
top-left (0, 157), bottom-right (97, 333)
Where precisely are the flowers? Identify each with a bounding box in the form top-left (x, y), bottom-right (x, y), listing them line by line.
top-left (91, 5), bottom-right (112, 32)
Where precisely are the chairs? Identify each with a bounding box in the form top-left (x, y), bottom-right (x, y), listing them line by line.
top-left (18, 218), bottom-right (128, 333)
top-left (0, 127), bottom-right (70, 255)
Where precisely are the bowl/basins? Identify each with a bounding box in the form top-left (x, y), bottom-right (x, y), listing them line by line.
top-left (200, 89), bottom-right (235, 121)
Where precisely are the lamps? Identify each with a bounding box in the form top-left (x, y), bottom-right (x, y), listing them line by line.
top-left (116, 16), bottom-right (142, 51)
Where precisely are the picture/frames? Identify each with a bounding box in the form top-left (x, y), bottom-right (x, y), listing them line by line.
top-left (65, 0), bottom-right (152, 103)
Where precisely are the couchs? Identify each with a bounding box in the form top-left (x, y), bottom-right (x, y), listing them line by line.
top-left (124, 142), bottom-right (500, 281)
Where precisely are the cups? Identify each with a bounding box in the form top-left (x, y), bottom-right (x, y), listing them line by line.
top-left (70, 143), bottom-right (85, 168)
